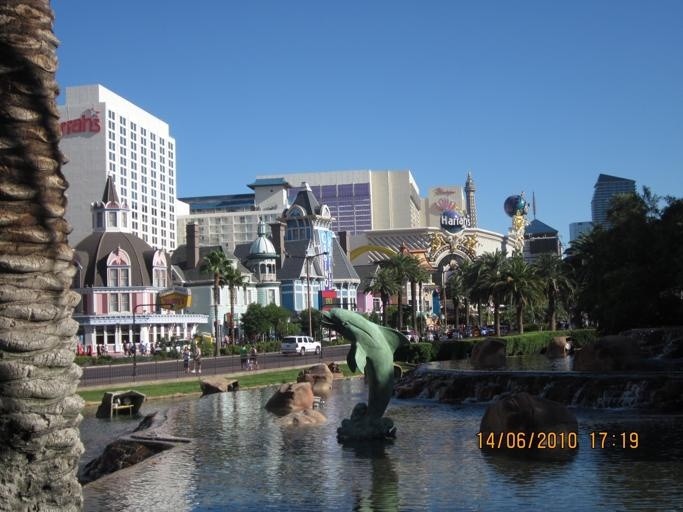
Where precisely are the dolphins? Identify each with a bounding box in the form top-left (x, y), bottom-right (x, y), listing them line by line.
top-left (318, 306), bottom-right (411, 437)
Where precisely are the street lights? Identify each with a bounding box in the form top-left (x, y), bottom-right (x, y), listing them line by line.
top-left (320, 304), bottom-right (357, 338)
top-left (306, 251), bottom-right (329, 337)
top-left (132, 304), bottom-right (175, 376)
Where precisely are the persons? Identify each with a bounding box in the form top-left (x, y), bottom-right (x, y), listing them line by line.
top-left (562, 335), bottom-right (583, 360)
top-left (122, 334), bottom-right (259, 375)
top-left (95, 342), bottom-right (109, 357)
top-left (404, 322), bottom-right (494, 344)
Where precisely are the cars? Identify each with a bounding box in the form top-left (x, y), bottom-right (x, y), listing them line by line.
top-left (280, 336), bottom-right (321, 357)
top-left (399, 324), bottom-right (508, 342)
